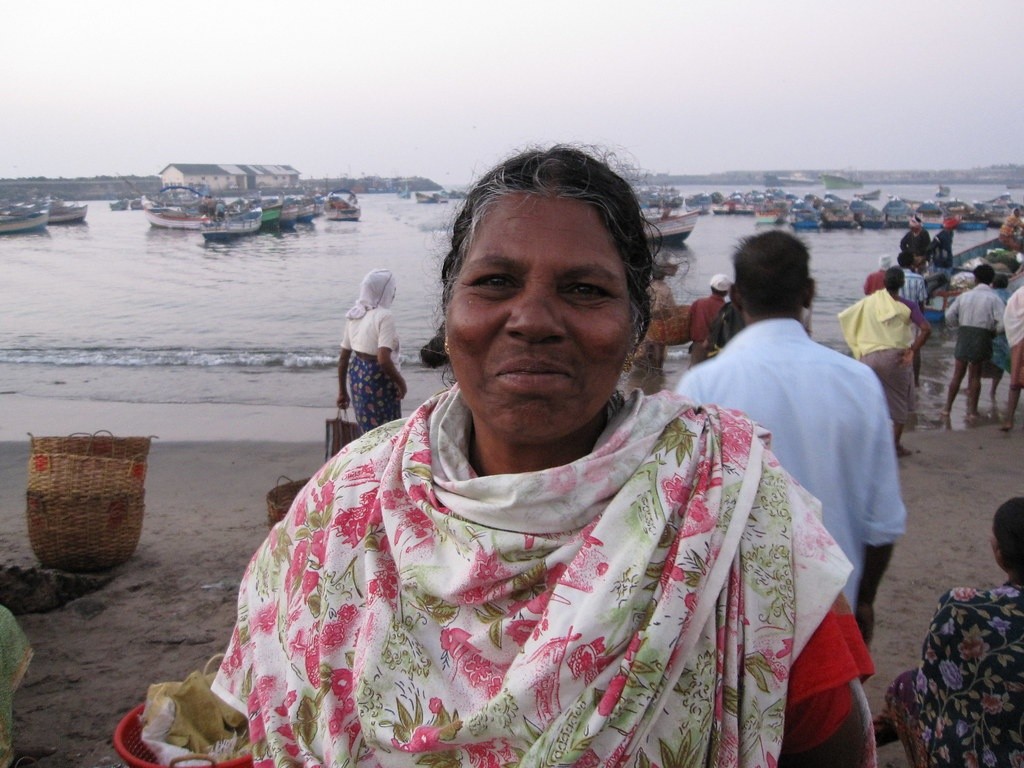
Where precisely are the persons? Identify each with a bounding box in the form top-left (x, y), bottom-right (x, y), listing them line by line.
top-left (210, 144), bottom-right (875, 768)
top-left (939, 263), bottom-right (1024, 430)
top-left (999, 207), bottom-right (1024, 253)
top-left (854, 267), bottom-right (932, 458)
top-left (864, 213), bottom-right (958, 387)
top-left (686, 273), bottom-right (746, 370)
top-left (673, 230), bottom-right (908, 649)
top-left (871, 496), bottom-right (1024, 768)
top-left (336, 270), bottom-right (407, 436)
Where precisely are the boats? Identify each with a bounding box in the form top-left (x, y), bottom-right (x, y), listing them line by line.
top-left (107, 183), bottom-right (362, 243)
top-left (915, 231), bottom-right (1024, 324)
top-left (0, 193), bottom-right (89, 235)
top-left (631, 181), bottom-right (1024, 236)
top-left (398, 184), bottom-right (470, 204)
top-left (640, 205), bottom-right (701, 246)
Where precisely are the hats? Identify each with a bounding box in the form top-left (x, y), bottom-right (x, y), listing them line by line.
top-left (709, 275), bottom-right (733, 292)
top-left (879, 254), bottom-right (892, 270)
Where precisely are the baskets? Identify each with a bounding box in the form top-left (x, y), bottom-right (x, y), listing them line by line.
top-left (114, 702), bottom-right (257, 768)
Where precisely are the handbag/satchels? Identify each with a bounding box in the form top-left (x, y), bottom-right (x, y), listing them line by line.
top-left (324, 406), bottom-right (363, 467)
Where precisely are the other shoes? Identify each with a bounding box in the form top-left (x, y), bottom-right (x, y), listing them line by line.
top-left (895, 446), bottom-right (912, 458)
